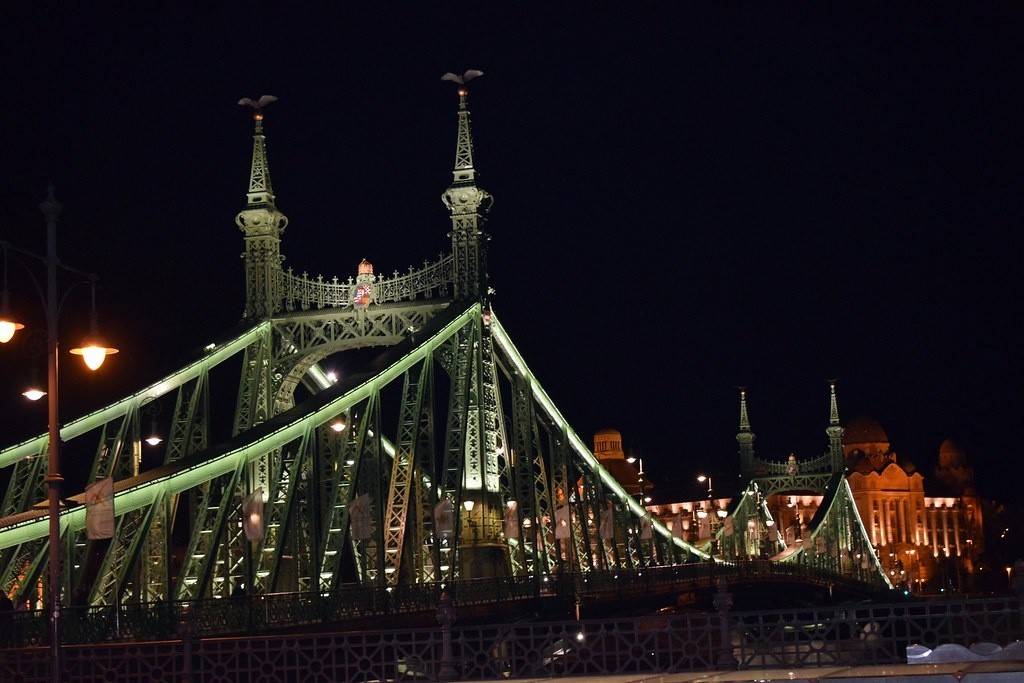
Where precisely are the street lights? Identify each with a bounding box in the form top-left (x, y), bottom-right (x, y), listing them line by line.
top-left (697, 473), bottom-right (713, 508)
top-left (626, 456), bottom-right (644, 506)
top-left (0, 179), bottom-right (120, 683)
